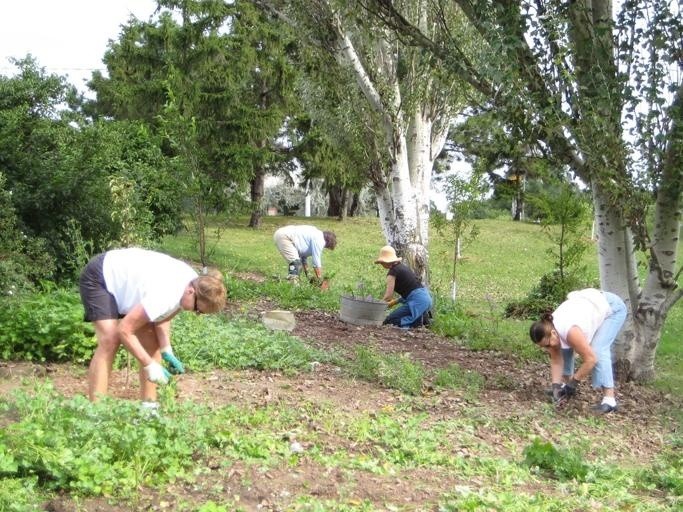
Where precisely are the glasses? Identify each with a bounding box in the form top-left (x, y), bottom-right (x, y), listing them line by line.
top-left (193, 295), bottom-right (203, 316)
top-left (540, 337), bottom-right (552, 349)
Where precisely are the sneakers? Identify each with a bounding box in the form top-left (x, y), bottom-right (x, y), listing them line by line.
top-left (591, 402), bottom-right (619, 415)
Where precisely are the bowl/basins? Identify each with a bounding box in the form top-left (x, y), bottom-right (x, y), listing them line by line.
top-left (340, 294), bottom-right (390, 328)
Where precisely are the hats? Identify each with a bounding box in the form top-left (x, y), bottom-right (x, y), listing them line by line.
top-left (374, 244), bottom-right (402, 264)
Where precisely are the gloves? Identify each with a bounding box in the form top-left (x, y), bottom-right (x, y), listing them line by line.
top-left (143, 359), bottom-right (172, 383)
top-left (160, 347), bottom-right (184, 375)
top-left (562, 377), bottom-right (580, 399)
top-left (550, 382), bottom-right (566, 401)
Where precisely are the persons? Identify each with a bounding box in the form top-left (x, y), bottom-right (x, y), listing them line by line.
top-left (274, 225), bottom-right (337, 288)
top-left (374, 245), bottom-right (433, 328)
top-left (79, 248), bottom-right (226, 421)
top-left (530, 288), bottom-right (628, 413)
